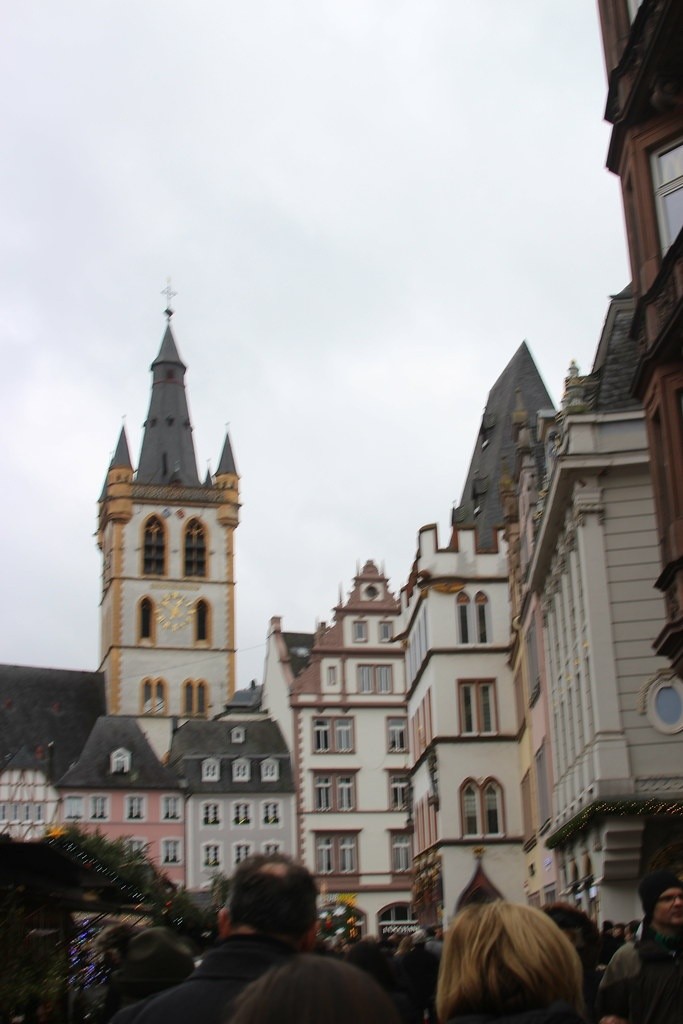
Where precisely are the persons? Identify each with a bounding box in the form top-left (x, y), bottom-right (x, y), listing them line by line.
top-left (436, 900), bottom-right (584, 1024)
top-left (110, 851), bottom-right (322, 1024)
top-left (597, 870), bottom-right (683, 1024)
top-left (100, 923), bottom-right (195, 1024)
top-left (317, 930), bottom-right (442, 1024)
top-left (231, 950), bottom-right (401, 1024)
top-left (540, 903), bottom-right (640, 1024)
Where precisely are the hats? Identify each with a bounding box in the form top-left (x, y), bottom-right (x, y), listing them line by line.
top-left (112, 926), bottom-right (194, 989)
top-left (637, 870), bottom-right (683, 917)
